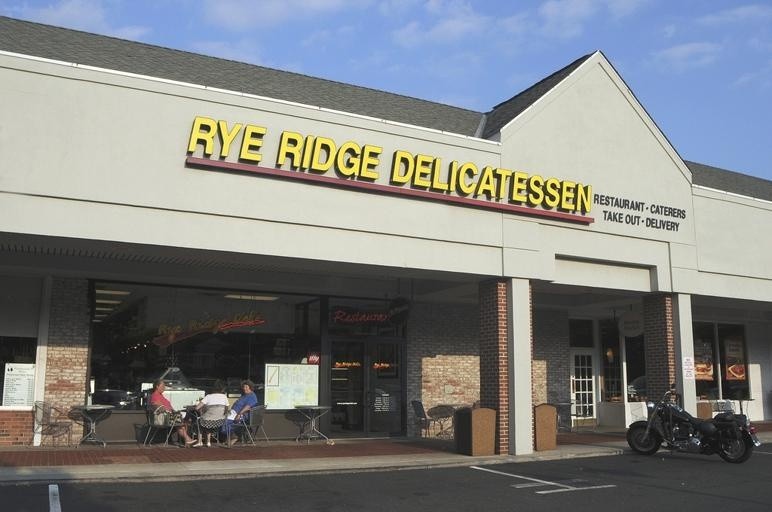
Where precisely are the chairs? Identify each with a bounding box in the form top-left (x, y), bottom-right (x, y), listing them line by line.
top-left (143, 403), bottom-right (270, 447)
top-left (411, 401), bottom-right (438, 439)
top-left (34, 401), bottom-right (71, 448)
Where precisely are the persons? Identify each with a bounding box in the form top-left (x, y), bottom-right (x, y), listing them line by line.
top-left (148, 379), bottom-right (197, 445)
top-left (219, 379), bottom-right (258, 445)
top-left (192, 378), bottom-right (230, 448)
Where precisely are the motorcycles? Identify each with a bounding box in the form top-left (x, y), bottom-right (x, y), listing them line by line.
top-left (626, 384), bottom-right (761, 464)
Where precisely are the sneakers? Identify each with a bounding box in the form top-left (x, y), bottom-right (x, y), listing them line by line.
top-left (186, 438), bottom-right (211, 448)
top-left (222, 435), bottom-right (237, 446)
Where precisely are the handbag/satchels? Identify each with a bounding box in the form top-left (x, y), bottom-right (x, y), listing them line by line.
top-left (153, 413), bottom-right (165, 426)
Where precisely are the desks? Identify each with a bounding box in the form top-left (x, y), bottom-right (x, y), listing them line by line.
top-left (427, 405), bottom-right (456, 439)
top-left (296, 405), bottom-right (332, 445)
top-left (71, 404), bottom-right (115, 448)
top-left (555, 402), bottom-right (572, 435)
